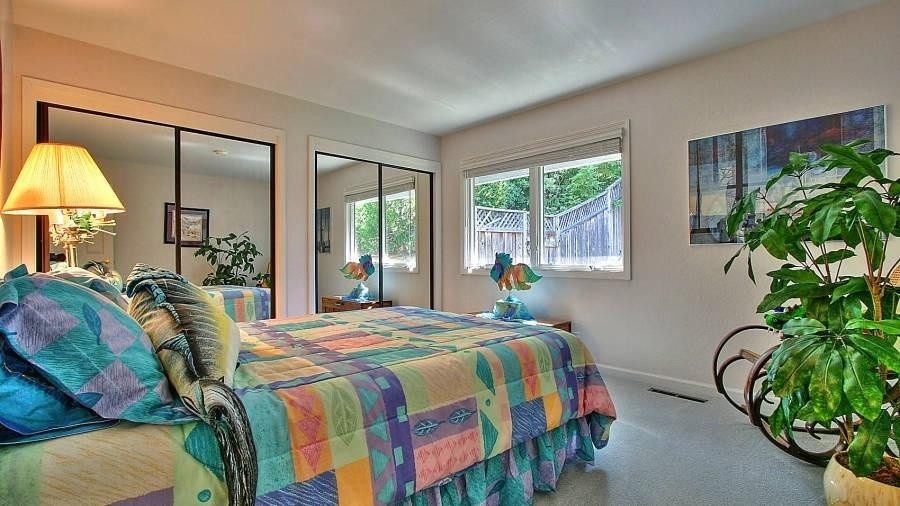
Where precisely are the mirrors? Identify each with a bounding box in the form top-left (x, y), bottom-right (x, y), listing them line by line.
top-left (20, 73), bottom-right (288, 326)
top-left (308, 133), bottom-right (444, 315)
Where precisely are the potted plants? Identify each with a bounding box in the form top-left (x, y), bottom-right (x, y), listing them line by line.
top-left (716, 135), bottom-right (900, 506)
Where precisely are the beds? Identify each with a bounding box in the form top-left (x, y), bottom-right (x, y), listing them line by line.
top-left (120, 285), bottom-right (272, 323)
top-left (0, 303), bottom-right (584, 506)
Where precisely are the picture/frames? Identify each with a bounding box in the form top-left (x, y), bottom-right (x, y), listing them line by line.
top-left (163, 201), bottom-right (211, 249)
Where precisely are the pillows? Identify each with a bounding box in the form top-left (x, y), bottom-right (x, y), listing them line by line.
top-left (81, 260), bottom-right (124, 291)
top-left (1, 331), bottom-right (111, 438)
top-left (1, 263), bottom-right (201, 425)
top-left (43, 267), bottom-right (130, 310)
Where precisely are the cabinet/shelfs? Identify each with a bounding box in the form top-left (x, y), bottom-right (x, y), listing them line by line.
top-left (320, 295), bottom-right (393, 312)
top-left (468, 311), bottom-right (571, 333)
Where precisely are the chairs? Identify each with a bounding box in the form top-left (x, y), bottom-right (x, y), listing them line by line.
top-left (712, 260), bottom-right (900, 462)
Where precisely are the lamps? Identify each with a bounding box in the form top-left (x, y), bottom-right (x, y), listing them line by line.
top-left (2, 142), bottom-right (127, 268)
